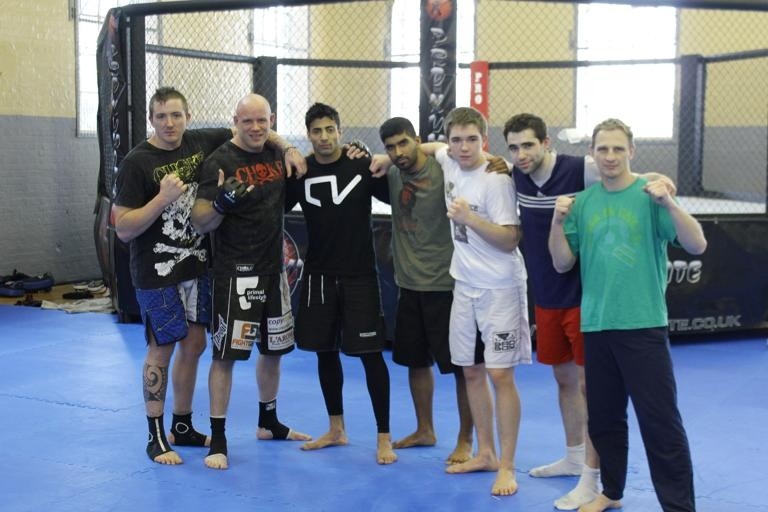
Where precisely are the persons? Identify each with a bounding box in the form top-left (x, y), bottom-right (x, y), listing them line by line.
top-left (545, 119), bottom-right (708, 511)
top-left (378, 113), bottom-right (513, 464)
top-left (367, 105), bottom-right (533, 495)
top-left (282, 98), bottom-right (402, 464)
top-left (498, 113), bottom-right (679, 510)
top-left (189, 94), bottom-right (372, 468)
top-left (114, 88), bottom-right (307, 464)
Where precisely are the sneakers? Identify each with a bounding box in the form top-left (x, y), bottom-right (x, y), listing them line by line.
top-left (62, 280), bottom-right (106, 299)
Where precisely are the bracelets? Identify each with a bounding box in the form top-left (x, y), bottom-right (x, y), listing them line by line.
top-left (281, 143), bottom-right (296, 157)
